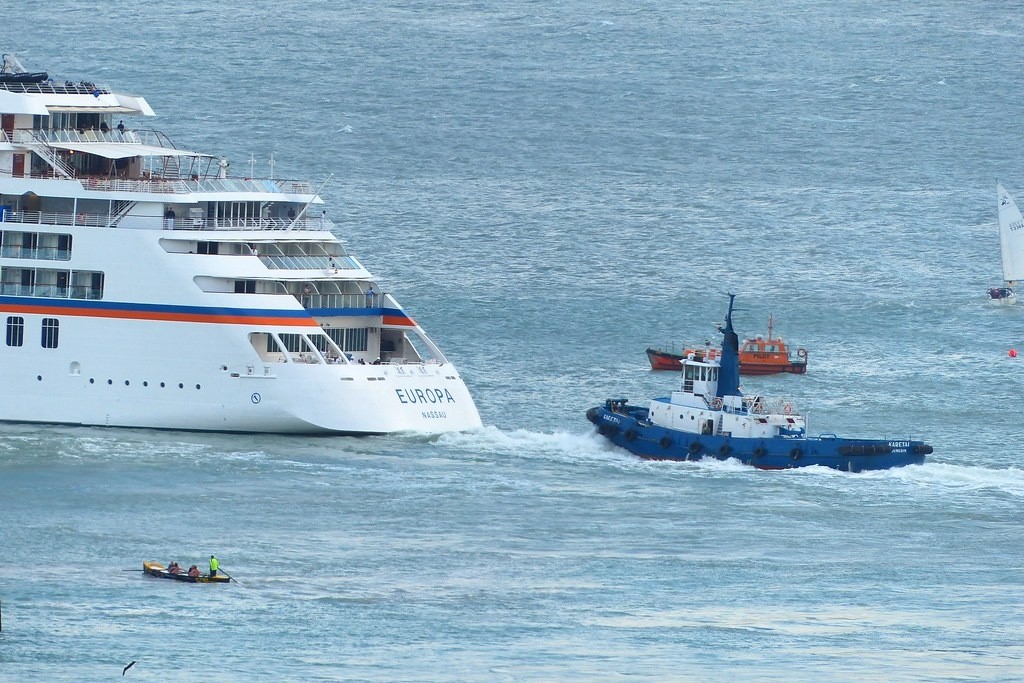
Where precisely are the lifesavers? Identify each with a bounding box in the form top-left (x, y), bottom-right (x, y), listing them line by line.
top-left (661, 437), bottom-right (670, 448)
top-left (88, 177), bottom-right (98, 187)
top-left (798, 349), bottom-right (806, 357)
top-left (784, 404), bottom-right (792, 415)
top-left (625, 430), bottom-right (636, 440)
top-left (607, 425), bottom-right (616, 435)
top-left (599, 424), bottom-right (608, 435)
top-left (714, 399), bottom-right (722, 408)
top-left (754, 447), bottom-right (766, 457)
top-left (790, 448), bottom-right (802, 460)
top-left (78, 213), bottom-right (86, 222)
top-left (690, 443), bottom-right (701, 453)
top-left (720, 446), bottom-right (730, 455)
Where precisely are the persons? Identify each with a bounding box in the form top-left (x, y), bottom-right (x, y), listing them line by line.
top-left (60, 274), bottom-right (66, 298)
top-left (188, 565), bottom-right (199, 577)
top-left (100, 119), bottom-right (109, 134)
top-left (117, 120), bottom-right (124, 135)
top-left (702, 423), bottom-right (710, 435)
top-left (705, 339), bottom-right (711, 349)
top-left (365, 286), bottom-right (377, 308)
top-left (320, 210), bottom-right (326, 230)
top-left (168, 561), bottom-right (179, 573)
top-left (302, 284), bottom-right (311, 308)
top-left (165, 207), bottom-right (175, 230)
top-left (208, 555), bottom-right (219, 577)
top-left (251, 243), bottom-right (259, 257)
top-left (287, 207), bottom-right (295, 230)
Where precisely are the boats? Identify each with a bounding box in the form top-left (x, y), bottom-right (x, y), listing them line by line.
top-left (0, 49), bottom-right (483, 438)
top-left (645, 311), bottom-right (808, 376)
top-left (588, 290), bottom-right (933, 470)
top-left (143, 560), bottom-right (232, 583)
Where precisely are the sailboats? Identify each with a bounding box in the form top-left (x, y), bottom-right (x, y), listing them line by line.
top-left (985, 177), bottom-right (1024, 308)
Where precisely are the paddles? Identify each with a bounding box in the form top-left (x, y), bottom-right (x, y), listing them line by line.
top-left (217, 567), bottom-right (237, 583)
